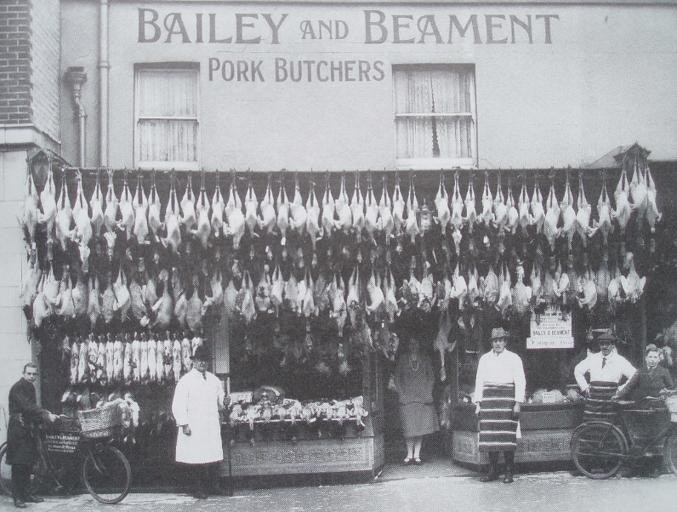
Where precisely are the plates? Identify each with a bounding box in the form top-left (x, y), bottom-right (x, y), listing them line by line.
top-left (145, 409), bottom-right (387, 483)
top-left (452, 398), bottom-right (583, 475)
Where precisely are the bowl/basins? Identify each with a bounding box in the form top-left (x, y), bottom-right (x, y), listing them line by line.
top-left (407, 351), bottom-right (421, 372)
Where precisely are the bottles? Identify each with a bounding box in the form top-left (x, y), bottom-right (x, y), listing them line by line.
top-left (488, 327), bottom-right (507, 341)
top-left (189, 345), bottom-right (215, 362)
top-left (595, 335), bottom-right (616, 343)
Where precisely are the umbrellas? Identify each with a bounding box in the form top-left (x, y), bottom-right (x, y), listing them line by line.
top-left (14, 498), bottom-right (26, 507)
top-left (21, 494), bottom-right (44, 502)
top-left (190, 487), bottom-right (232, 499)
top-left (503, 472), bottom-right (512, 483)
top-left (480, 470), bottom-right (498, 482)
top-left (402, 457), bottom-right (422, 465)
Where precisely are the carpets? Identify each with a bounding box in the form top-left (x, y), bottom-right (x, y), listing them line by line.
top-left (600, 357), bottom-right (606, 369)
top-left (202, 374), bottom-right (206, 380)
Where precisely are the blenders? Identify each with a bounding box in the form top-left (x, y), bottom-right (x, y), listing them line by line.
top-left (229, 395), bottom-right (369, 449)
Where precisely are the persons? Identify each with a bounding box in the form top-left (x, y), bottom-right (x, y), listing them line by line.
top-left (471, 325), bottom-right (527, 485)
top-left (392, 329), bottom-right (441, 465)
top-left (611, 343), bottom-right (676, 479)
top-left (568, 334), bottom-right (641, 478)
top-left (171, 344), bottom-right (233, 499)
top-left (5, 362), bottom-right (62, 510)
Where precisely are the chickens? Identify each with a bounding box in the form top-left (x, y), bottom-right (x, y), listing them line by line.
top-left (229, 395), bottom-right (369, 449)
top-left (15, 151), bottom-right (663, 382)
